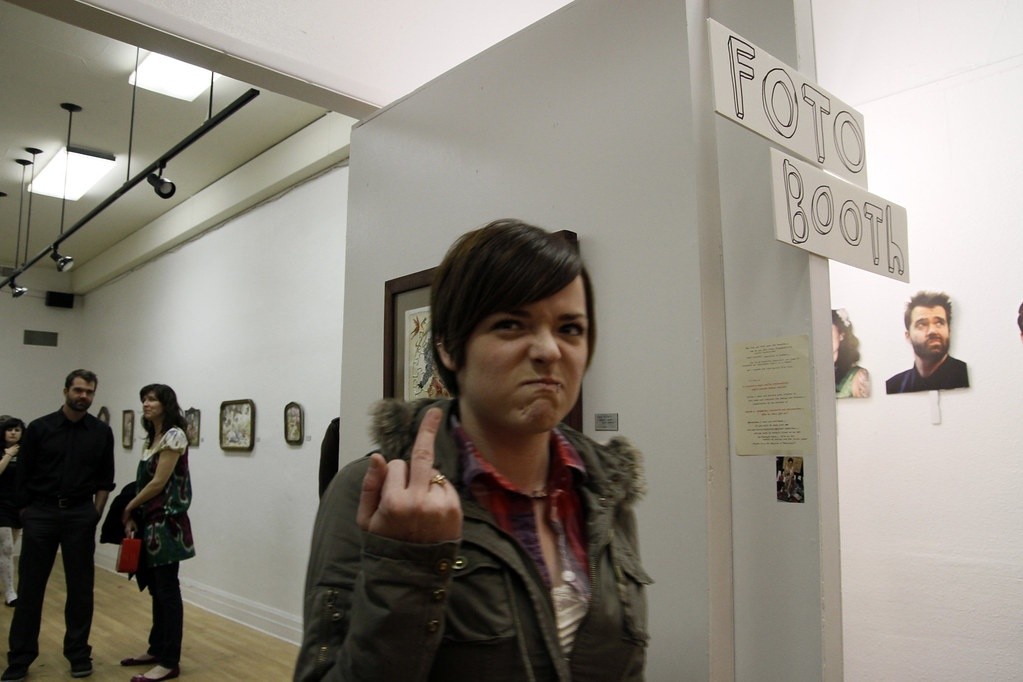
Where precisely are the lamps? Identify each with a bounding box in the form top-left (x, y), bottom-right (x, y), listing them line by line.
top-left (28, 145), bottom-right (116, 201)
top-left (10, 277), bottom-right (29, 300)
top-left (50, 249), bottom-right (76, 273)
top-left (146, 162), bottom-right (176, 198)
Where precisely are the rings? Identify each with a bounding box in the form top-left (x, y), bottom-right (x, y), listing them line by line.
top-left (429, 475), bottom-right (447, 487)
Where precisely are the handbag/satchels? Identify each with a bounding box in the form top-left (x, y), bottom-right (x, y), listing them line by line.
top-left (116, 531), bottom-right (141, 573)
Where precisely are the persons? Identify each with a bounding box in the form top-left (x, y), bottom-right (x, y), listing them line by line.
top-left (831, 309), bottom-right (870, 401)
top-left (120, 383), bottom-right (195, 682)
top-left (1, 414), bottom-right (28, 606)
top-left (289, 219), bottom-right (658, 682)
top-left (1, 369), bottom-right (115, 682)
top-left (883, 291), bottom-right (970, 395)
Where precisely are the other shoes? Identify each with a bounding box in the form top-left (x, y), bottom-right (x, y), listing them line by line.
top-left (71, 654), bottom-right (92, 677)
top-left (120, 653), bottom-right (157, 666)
top-left (1, 665), bottom-right (28, 682)
top-left (131, 665), bottom-right (180, 682)
top-left (5, 597), bottom-right (17, 607)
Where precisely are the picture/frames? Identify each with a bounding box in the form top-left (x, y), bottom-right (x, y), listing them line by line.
top-left (218, 399), bottom-right (257, 450)
top-left (283, 402), bottom-right (305, 446)
top-left (185, 406), bottom-right (201, 446)
top-left (122, 410), bottom-right (134, 448)
top-left (383, 230), bottom-right (584, 434)
